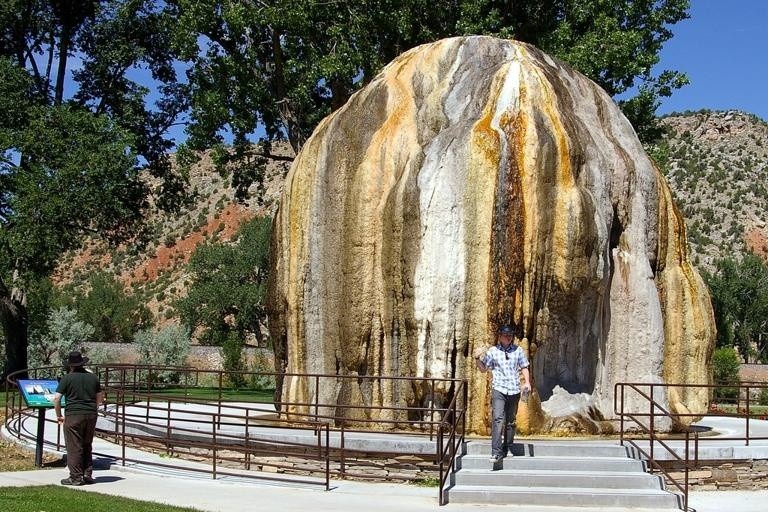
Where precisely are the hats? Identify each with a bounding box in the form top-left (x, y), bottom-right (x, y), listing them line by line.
top-left (62, 352), bottom-right (89, 366)
top-left (501, 326), bottom-right (512, 332)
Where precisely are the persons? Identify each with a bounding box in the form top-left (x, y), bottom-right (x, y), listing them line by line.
top-left (54, 351), bottom-right (102, 486)
top-left (471, 325), bottom-right (531, 463)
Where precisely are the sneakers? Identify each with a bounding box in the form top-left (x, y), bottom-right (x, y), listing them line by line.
top-left (83, 475), bottom-right (95, 484)
top-left (490, 454), bottom-right (504, 463)
top-left (61, 478), bottom-right (85, 485)
top-left (505, 447), bottom-right (514, 457)
